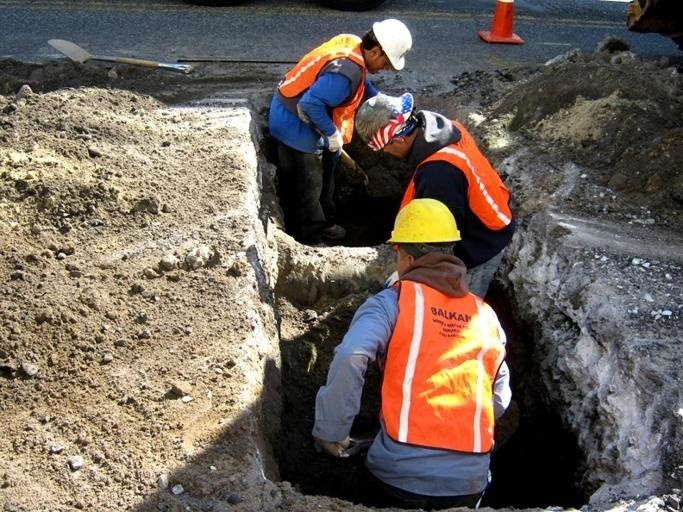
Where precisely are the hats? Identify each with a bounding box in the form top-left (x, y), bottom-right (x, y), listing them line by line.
top-left (354, 92), bottom-right (414, 153)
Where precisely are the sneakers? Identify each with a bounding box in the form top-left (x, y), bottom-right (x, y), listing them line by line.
top-left (323, 223), bottom-right (347, 239)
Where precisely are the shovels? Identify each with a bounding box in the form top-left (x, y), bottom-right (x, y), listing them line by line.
top-left (314, 400), bottom-right (520, 458)
top-left (297, 104), bottom-right (403, 197)
top-left (48, 38), bottom-right (192, 74)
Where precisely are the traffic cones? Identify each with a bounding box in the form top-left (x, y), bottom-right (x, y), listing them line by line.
top-left (479, 0), bottom-right (524, 44)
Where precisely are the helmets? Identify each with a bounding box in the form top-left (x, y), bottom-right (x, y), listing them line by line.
top-left (384, 197), bottom-right (463, 245)
top-left (372, 18), bottom-right (413, 71)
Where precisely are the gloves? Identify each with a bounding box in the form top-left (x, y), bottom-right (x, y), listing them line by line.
top-left (316, 435), bottom-right (350, 458)
top-left (323, 126), bottom-right (344, 158)
top-left (384, 270), bottom-right (399, 288)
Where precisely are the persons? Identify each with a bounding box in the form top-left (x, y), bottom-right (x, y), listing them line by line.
top-left (268, 19), bottom-right (409, 244)
top-left (311, 199), bottom-right (516, 506)
top-left (350, 93), bottom-right (512, 304)
